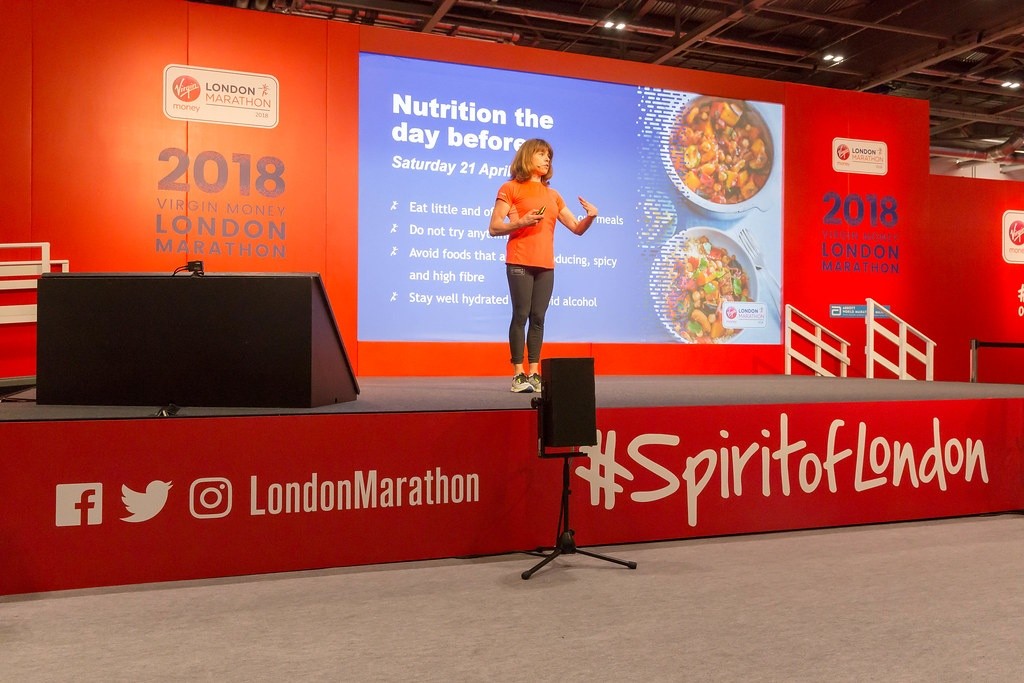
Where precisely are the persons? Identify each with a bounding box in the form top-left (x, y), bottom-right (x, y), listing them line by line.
top-left (488, 138), bottom-right (598, 393)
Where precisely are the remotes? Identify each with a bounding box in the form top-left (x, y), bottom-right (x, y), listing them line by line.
top-left (537, 205), bottom-right (546, 215)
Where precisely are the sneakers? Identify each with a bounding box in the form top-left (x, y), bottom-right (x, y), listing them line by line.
top-left (528, 373), bottom-right (541, 392)
top-left (510, 372), bottom-right (535, 393)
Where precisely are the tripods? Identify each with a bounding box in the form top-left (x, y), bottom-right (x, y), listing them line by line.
top-left (520, 458), bottom-right (638, 579)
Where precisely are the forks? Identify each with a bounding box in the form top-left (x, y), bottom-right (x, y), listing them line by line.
top-left (739, 228), bottom-right (781, 291)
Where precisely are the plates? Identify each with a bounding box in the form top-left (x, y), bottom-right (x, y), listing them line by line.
top-left (649, 226), bottom-right (759, 344)
top-left (659, 98), bottom-right (779, 213)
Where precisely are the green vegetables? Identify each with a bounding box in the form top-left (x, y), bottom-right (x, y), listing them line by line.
top-left (686, 259), bottom-right (748, 333)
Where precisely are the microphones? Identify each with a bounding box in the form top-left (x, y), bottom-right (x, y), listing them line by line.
top-left (537, 165), bottom-right (541, 167)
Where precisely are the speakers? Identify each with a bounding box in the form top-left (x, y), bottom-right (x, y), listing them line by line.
top-left (540, 357), bottom-right (598, 447)
